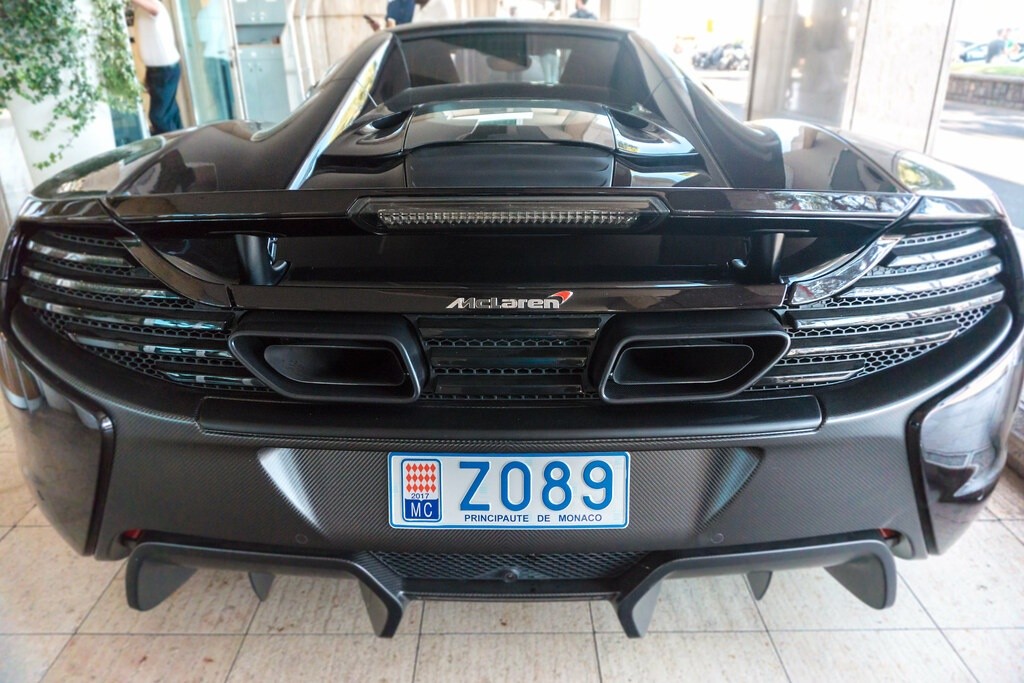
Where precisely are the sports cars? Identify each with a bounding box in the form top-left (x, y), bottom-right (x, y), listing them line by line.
top-left (0, 18), bottom-right (1024, 638)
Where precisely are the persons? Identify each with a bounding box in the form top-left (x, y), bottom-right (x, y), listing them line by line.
top-left (196, 1), bottom-right (237, 119)
top-left (567, 0), bottom-right (598, 23)
top-left (129, 0), bottom-right (184, 135)
top-left (385, 0), bottom-right (429, 31)
